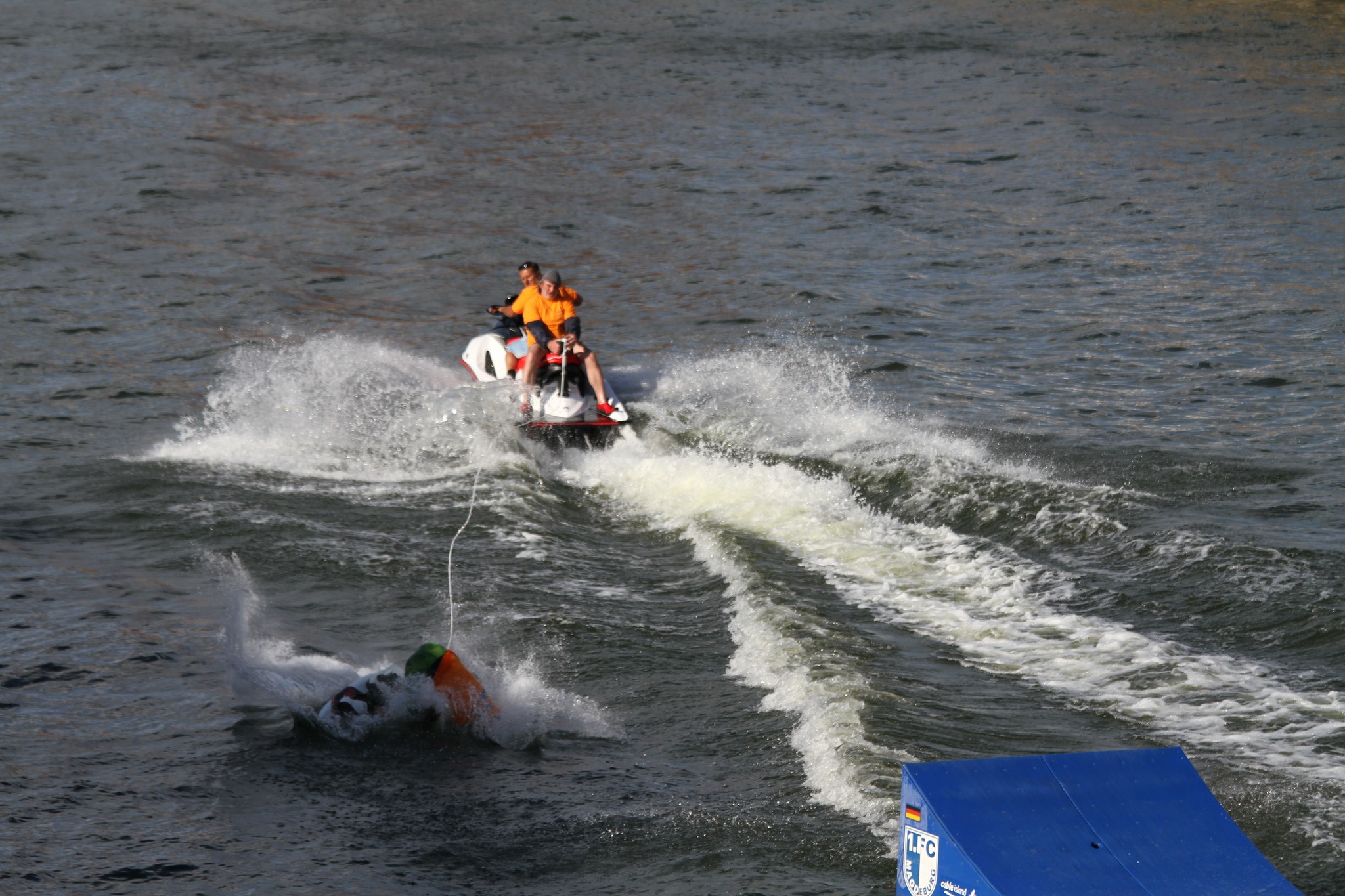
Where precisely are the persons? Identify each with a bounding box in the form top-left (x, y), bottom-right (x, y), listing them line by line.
top-left (517, 270), bottom-right (618, 420)
top-left (405, 642), bottom-right (500, 726)
top-left (488, 261), bottom-right (583, 384)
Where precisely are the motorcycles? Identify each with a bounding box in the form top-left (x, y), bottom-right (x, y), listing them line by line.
top-left (460, 294), bottom-right (630, 429)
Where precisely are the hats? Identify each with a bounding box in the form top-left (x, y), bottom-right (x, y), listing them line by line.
top-left (541, 270), bottom-right (563, 285)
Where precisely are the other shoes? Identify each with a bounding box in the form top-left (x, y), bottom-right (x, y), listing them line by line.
top-left (521, 404), bottom-right (533, 420)
top-left (597, 403), bottom-right (618, 414)
